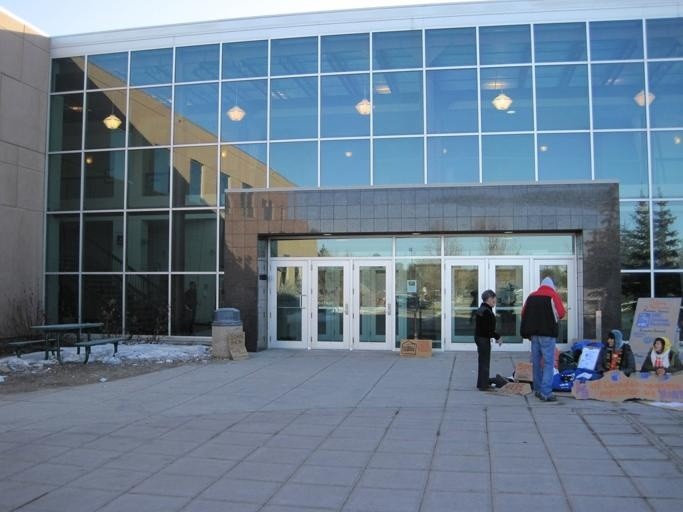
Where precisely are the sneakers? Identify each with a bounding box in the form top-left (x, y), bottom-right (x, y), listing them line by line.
top-left (534, 391), bottom-right (558, 402)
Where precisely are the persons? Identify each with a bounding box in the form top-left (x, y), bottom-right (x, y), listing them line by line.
top-left (596, 329), bottom-right (635, 378)
top-left (467, 291), bottom-right (476, 322)
top-left (640, 336), bottom-right (683, 377)
top-left (518, 277), bottom-right (566, 401)
top-left (496, 282), bottom-right (516, 335)
top-left (180, 280), bottom-right (201, 336)
top-left (474, 289), bottom-right (503, 391)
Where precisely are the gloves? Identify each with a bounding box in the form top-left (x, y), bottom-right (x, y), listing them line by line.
top-left (496, 337), bottom-right (503, 346)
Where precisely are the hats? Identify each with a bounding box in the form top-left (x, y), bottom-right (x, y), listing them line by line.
top-left (541, 277), bottom-right (554, 289)
top-left (481, 290), bottom-right (495, 300)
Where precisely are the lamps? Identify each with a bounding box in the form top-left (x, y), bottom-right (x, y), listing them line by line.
top-left (102, 90), bottom-right (121, 131)
top-left (634, 63), bottom-right (655, 107)
top-left (227, 87), bottom-right (245, 123)
top-left (354, 73), bottom-right (371, 116)
top-left (491, 67), bottom-right (512, 112)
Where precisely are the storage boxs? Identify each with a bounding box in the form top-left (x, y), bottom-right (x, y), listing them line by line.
top-left (400, 339), bottom-right (432, 357)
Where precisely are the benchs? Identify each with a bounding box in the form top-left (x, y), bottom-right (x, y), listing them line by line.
top-left (10, 338), bottom-right (57, 360)
top-left (75, 337), bottom-right (125, 364)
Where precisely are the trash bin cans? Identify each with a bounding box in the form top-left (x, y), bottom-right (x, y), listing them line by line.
top-left (211, 308), bottom-right (243, 357)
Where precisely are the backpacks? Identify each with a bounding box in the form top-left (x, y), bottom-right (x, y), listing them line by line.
top-left (552, 340), bottom-right (605, 391)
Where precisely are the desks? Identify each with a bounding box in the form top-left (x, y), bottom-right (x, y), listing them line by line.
top-left (30, 323), bottom-right (103, 362)
